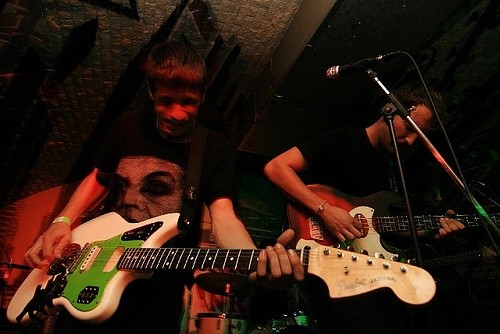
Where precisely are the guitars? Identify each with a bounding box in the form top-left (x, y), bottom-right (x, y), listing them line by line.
top-left (285, 184), bottom-right (500, 267)
top-left (5, 209), bottom-right (437, 326)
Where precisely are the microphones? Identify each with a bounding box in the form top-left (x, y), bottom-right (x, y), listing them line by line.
top-left (327, 51), bottom-right (401, 82)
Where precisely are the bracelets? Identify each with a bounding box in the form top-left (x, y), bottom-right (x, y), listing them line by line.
top-left (314, 199), bottom-right (327, 215)
top-left (52, 217), bottom-right (71, 224)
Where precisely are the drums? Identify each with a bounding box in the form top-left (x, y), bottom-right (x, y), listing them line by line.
top-left (196, 312), bottom-right (253, 333)
top-left (269, 310), bottom-right (315, 331)
top-left (250, 325), bottom-right (276, 334)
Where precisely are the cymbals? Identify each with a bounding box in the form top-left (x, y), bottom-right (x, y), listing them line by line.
top-left (195, 272), bottom-right (262, 298)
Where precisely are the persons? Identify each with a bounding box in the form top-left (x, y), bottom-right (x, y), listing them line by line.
top-left (265, 82), bottom-right (466, 334)
top-left (25, 40), bottom-right (304, 334)
